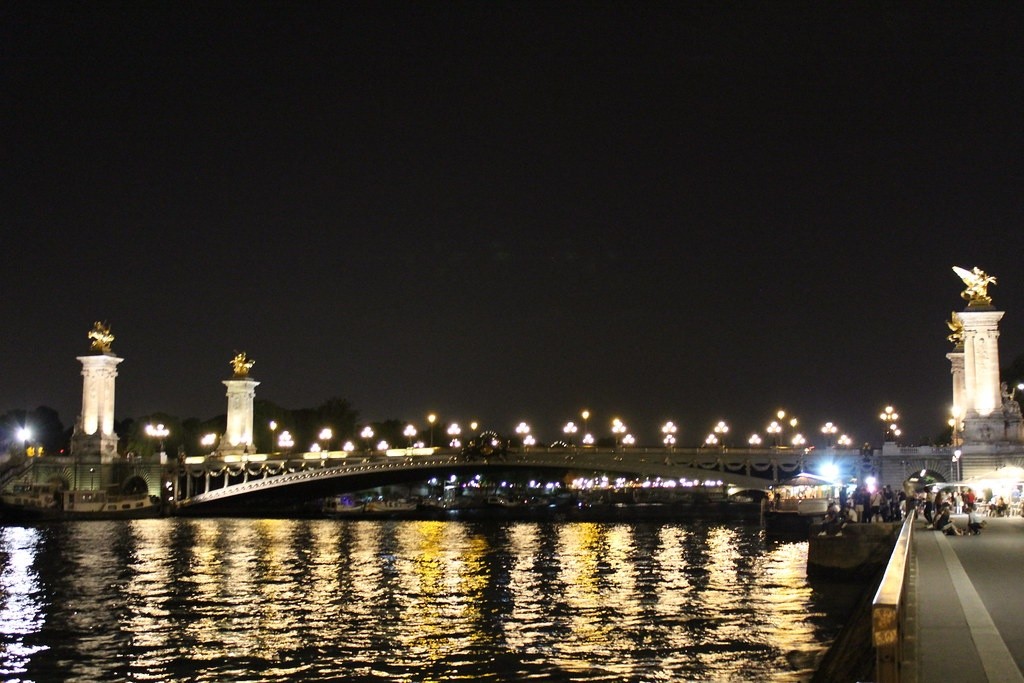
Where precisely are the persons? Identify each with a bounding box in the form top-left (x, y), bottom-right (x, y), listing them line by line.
top-left (818, 508), bottom-right (837, 536)
top-left (932, 489), bottom-right (1011, 535)
top-left (923, 487), bottom-right (934, 528)
top-left (836, 505), bottom-right (858, 536)
top-left (827, 484), bottom-right (925, 523)
